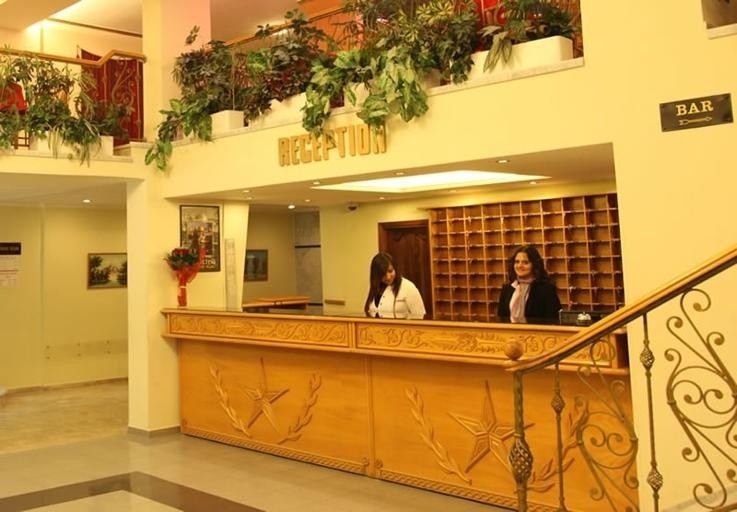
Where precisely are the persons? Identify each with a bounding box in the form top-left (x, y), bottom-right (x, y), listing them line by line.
top-left (364, 252), bottom-right (426, 319)
top-left (495, 245), bottom-right (562, 318)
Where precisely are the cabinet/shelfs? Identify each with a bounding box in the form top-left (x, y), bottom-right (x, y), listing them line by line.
top-left (413, 191), bottom-right (627, 323)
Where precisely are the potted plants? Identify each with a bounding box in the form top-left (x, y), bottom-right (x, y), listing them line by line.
top-left (1, 44), bottom-right (123, 163)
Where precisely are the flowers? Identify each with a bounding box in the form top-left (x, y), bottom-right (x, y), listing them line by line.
top-left (164, 246), bottom-right (205, 282)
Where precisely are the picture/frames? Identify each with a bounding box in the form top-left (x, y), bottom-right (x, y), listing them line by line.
top-left (243, 248), bottom-right (270, 282)
top-left (178, 203), bottom-right (222, 274)
top-left (85, 251), bottom-right (127, 290)
